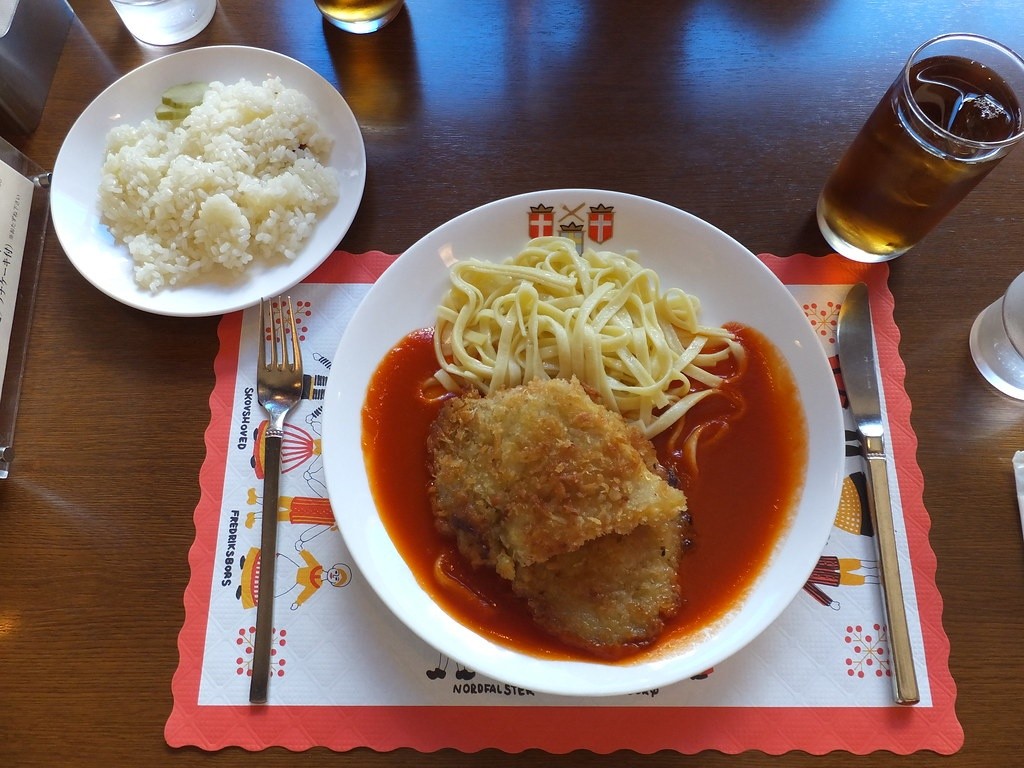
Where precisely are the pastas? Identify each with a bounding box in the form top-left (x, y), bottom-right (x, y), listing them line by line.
top-left (424, 233), bottom-right (751, 614)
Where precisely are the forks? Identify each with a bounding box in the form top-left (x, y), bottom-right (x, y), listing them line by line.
top-left (250, 295), bottom-right (304, 703)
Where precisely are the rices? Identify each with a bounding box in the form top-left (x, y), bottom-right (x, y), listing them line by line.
top-left (95, 74), bottom-right (338, 294)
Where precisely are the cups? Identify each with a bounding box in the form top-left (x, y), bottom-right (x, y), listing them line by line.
top-left (110, 0), bottom-right (217, 45)
top-left (313, 0), bottom-right (403, 34)
top-left (816, 32), bottom-right (1024, 264)
top-left (968, 271), bottom-right (1024, 401)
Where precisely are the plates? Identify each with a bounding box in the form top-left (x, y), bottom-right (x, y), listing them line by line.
top-left (50, 45), bottom-right (366, 317)
top-left (321, 189), bottom-right (845, 697)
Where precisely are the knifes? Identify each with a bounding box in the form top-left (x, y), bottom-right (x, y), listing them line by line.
top-left (836, 283), bottom-right (919, 705)
